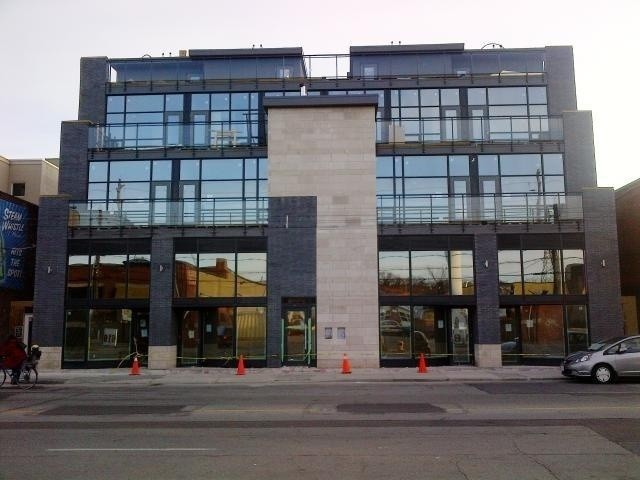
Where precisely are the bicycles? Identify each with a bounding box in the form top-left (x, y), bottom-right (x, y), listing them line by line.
top-left (0, 362), bottom-right (41, 390)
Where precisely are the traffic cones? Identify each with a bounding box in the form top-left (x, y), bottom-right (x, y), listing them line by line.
top-left (235, 354), bottom-right (246, 375)
top-left (417, 353), bottom-right (428, 373)
top-left (129, 355), bottom-right (140, 375)
top-left (341, 352), bottom-right (352, 373)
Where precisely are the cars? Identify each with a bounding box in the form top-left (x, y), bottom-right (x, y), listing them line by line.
top-left (561, 335), bottom-right (640, 383)
top-left (380, 319), bottom-right (403, 336)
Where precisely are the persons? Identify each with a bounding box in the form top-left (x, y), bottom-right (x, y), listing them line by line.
top-left (0, 334), bottom-right (27, 386)
